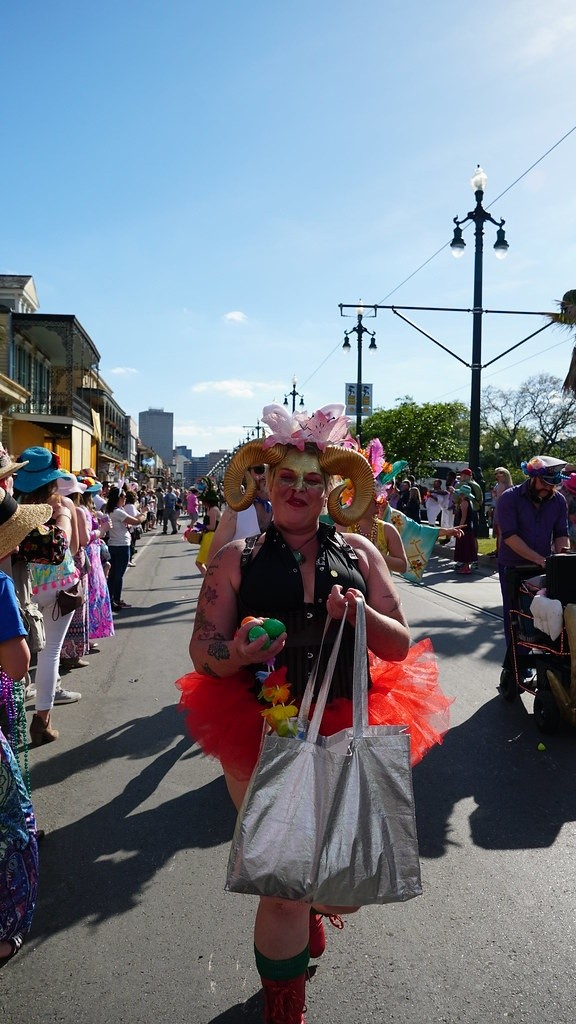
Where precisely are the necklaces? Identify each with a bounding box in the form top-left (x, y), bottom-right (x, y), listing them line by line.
top-left (355, 521), bottom-right (378, 543)
top-left (0, 670), bottom-right (31, 800)
top-left (288, 533), bottom-right (318, 564)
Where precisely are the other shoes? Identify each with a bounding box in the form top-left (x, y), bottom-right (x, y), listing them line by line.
top-left (118, 600), bottom-right (131, 609)
top-left (442, 542), bottom-right (455, 550)
top-left (88, 642), bottom-right (98, 647)
top-left (527, 669), bottom-right (533, 677)
top-left (54, 690), bottom-right (81, 703)
top-left (487, 551), bottom-right (498, 558)
top-left (127, 523), bottom-right (182, 567)
top-left (112, 602), bottom-right (121, 612)
top-left (69, 659), bottom-right (89, 667)
top-left (451, 560), bottom-right (480, 574)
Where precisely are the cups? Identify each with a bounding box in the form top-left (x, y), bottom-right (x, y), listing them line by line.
top-left (101, 514), bottom-right (110, 523)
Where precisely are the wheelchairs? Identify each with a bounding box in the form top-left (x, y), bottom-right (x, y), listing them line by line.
top-left (502, 551), bottom-right (576, 736)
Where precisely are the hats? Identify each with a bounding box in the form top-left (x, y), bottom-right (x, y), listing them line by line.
top-left (459, 469), bottom-right (473, 476)
top-left (0, 441), bottom-right (103, 560)
top-left (521, 455), bottom-right (571, 485)
top-left (454, 485), bottom-right (475, 498)
top-left (340, 438), bottom-right (409, 517)
top-left (195, 476), bottom-right (221, 502)
top-left (563, 473), bottom-right (576, 493)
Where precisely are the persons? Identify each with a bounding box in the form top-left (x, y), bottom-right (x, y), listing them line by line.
top-left (492, 455), bottom-right (576, 691)
top-left (388, 468), bottom-right (513, 574)
top-left (0, 441), bottom-right (228, 742)
top-left (333, 438), bottom-right (408, 575)
top-left (207, 459), bottom-right (274, 565)
top-left (175, 404), bottom-right (456, 1024)
top-left (0, 730), bottom-right (37, 958)
top-left (195, 490), bottom-right (223, 578)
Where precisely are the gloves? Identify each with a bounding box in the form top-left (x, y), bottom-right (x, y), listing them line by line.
top-left (194, 521), bottom-right (203, 529)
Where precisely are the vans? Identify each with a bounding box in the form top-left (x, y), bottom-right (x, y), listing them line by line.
top-left (418, 460), bottom-right (470, 494)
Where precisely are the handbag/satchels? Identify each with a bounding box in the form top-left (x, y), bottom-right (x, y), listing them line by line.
top-left (51, 580), bottom-right (85, 621)
top-left (19, 604), bottom-right (47, 652)
top-left (226, 596), bottom-right (424, 906)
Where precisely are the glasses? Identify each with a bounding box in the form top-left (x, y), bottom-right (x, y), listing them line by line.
top-left (248, 466), bottom-right (266, 475)
top-left (562, 470), bottom-right (575, 476)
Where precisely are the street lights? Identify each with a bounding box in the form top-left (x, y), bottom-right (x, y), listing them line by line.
top-left (534, 434), bottom-right (540, 456)
top-left (284, 373), bottom-right (306, 413)
top-left (513, 439), bottom-right (520, 469)
top-left (446, 162), bottom-right (513, 533)
top-left (494, 441), bottom-right (502, 468)
top-left (478, 444), bottom-right (484, 466)
top-left (342, 298), bottom-right (377, 452)
top-left (206, 416), bottom-right (264, 488)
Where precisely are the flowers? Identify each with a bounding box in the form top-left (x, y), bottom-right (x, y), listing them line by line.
top-left (521, 457), bottom-right (547, 477)
top-left (341, 447), bottom-right (391, 504)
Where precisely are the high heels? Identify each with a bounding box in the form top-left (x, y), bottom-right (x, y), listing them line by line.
top-left (30, 714), bottom-right (59, 744)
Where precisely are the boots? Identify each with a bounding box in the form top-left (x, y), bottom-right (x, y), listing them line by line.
top-left (258, 969), bottom-right (307, 1024)
top-left (309, 913), bottom-right (344, 958)
